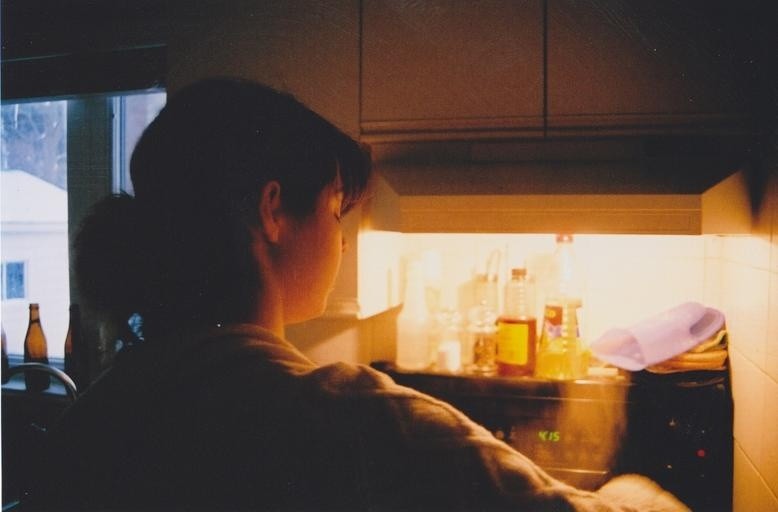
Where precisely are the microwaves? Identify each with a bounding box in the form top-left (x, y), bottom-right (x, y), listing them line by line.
top-left (374, 361), bottom-right (733, 512)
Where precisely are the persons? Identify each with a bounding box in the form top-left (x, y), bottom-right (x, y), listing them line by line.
top-left (19, 76), bottom-right (691, 512)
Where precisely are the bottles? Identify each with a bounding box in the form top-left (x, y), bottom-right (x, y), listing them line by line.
top-left (536, 230), bottom-right (584, 382)
top-left (24, 300), bottom-right (49, 393)
top-left (1, 326), bottom-right (11, 383)
top-left (496, 266), bottom-right (538, 381)
top-left (393, 257), bottom-right (499, 381)
top-left (64, 304), bottom-right (82, 387)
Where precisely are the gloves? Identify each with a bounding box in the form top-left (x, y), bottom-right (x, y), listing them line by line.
top-left (590, 301), bottom-right (725, 371)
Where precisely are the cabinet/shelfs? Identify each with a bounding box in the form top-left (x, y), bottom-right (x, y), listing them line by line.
top-left (165, 0), bottom-right (361, 147)
top-left (361, 0), bottom-right (777, 195)
